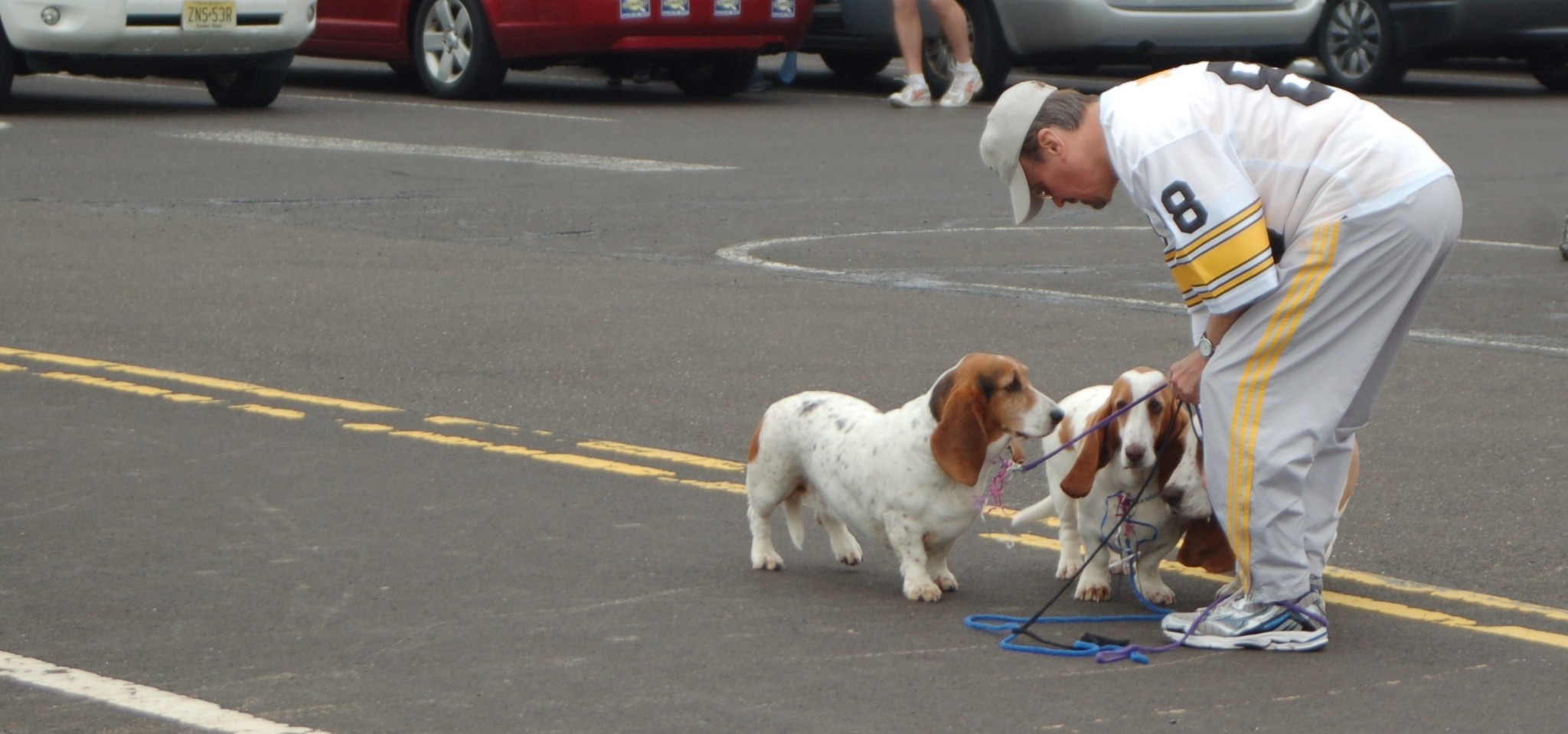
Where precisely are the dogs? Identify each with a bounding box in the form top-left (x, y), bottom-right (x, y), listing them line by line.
top-left (742, 350), bottom-right (1064, 599)
top-left (1010, 366), bottom-right (1194, 606)
top-left (1163, 410), bottom-right (1361, 603)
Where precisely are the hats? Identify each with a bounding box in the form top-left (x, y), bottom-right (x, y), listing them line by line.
top-left (979, 80), bottom-right (1060, 229)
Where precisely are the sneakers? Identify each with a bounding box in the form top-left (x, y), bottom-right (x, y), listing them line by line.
top-left (938, 57), bottom-right (984, 108)
top-left (1161, 582), bottom-right (1329, 652)
top-left (889, 73), bottom-right (931, 108)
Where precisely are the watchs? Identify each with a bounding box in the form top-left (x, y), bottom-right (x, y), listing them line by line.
top-left (1198, 331), bottom-right (1216, 357)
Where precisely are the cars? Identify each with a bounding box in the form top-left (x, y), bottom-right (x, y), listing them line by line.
top-left (293, 0), bottom-right (813, 100)
top-left (1299, 0), bottom-right (1568, 97)
top-left (760, 0), bottom-right (1327, 104)
top-left (0, 0), bottom-right (318, 109)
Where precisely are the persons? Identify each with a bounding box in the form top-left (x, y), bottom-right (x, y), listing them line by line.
top-left (978, 60), bottom-right (1461, 652)
top-left (888, 0), bottom-right (983, 107)
